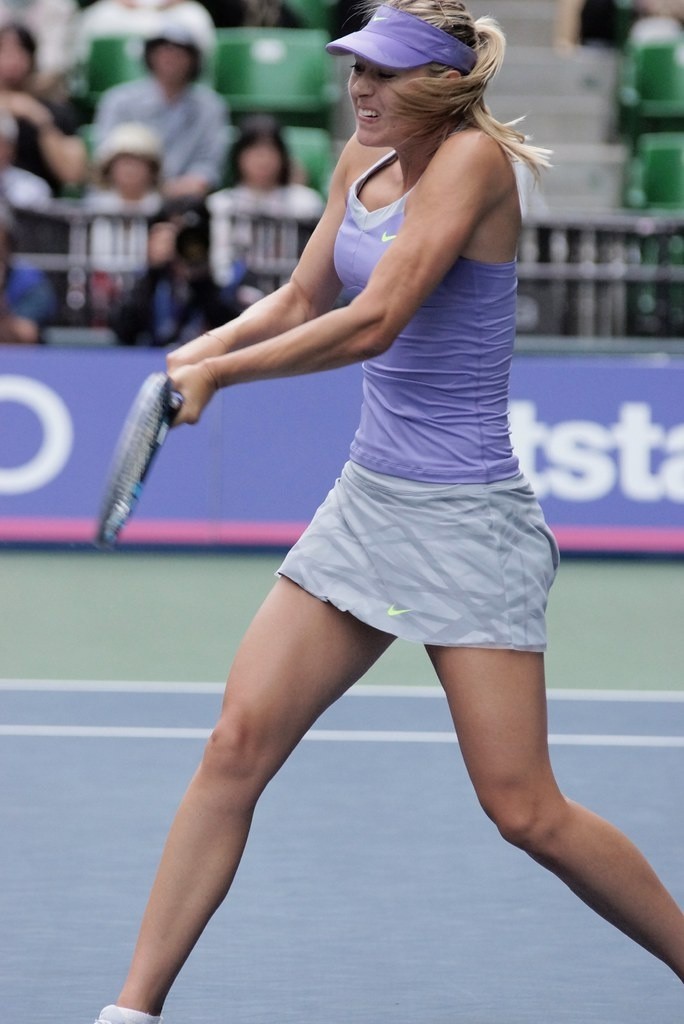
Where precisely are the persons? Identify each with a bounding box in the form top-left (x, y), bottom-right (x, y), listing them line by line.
top-left (0, 0), bottom-right (326, 346)
top-left (92, 0), bottom-right (684, 1024)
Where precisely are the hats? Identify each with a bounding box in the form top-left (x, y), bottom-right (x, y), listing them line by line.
top-left (96, 121), bottom-right (160, 169)
top-left (325, 4), bottom-right (477, 71)
top-left (143, 19), bottom-right (202, 82)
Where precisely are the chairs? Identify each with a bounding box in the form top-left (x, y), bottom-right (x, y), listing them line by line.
top-left (620, 44), bottom-right (684, 305)
top-left (66, 25), bottom-right (339, 200)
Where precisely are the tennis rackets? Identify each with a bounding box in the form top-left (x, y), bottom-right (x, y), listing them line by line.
top-left (96, 372), bottom-right (184, 545)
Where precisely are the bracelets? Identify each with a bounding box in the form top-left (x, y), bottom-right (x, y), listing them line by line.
top-left (201, 332), bottom-right (228, 354)
